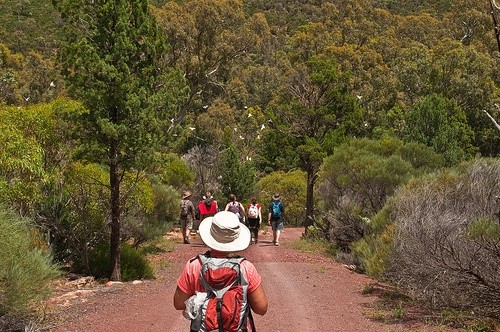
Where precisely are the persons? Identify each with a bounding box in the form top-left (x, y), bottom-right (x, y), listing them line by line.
top-left (178, 190), bottom-right (285, 246)
top-left (173, 210), bottom-right (268, 332)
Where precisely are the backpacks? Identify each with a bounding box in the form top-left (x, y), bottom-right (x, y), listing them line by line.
top-left (248, 204), bottom-right (259, 219)
top-left (190, 255), bottom-right (249, 332)
top-left (272, 202), bottom-right (281, 218)
top-left (181, 202), bottom-right (188, 216)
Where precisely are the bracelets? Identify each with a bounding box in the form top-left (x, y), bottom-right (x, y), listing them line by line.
top-left (268, 219), bottom-right (270, 221)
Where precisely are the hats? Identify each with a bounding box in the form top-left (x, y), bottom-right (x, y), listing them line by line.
top-left (182, 192), bottom-right (191, 199)
top-left (198, 211), bottom-right (251, 251)
top-left (272, 194), bottom-right (281, 200)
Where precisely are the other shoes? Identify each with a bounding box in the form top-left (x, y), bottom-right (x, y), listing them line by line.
top-left (275, 241), bottom-right (282, 246)
top-left (184, 237), bottom-right (190, 244)
